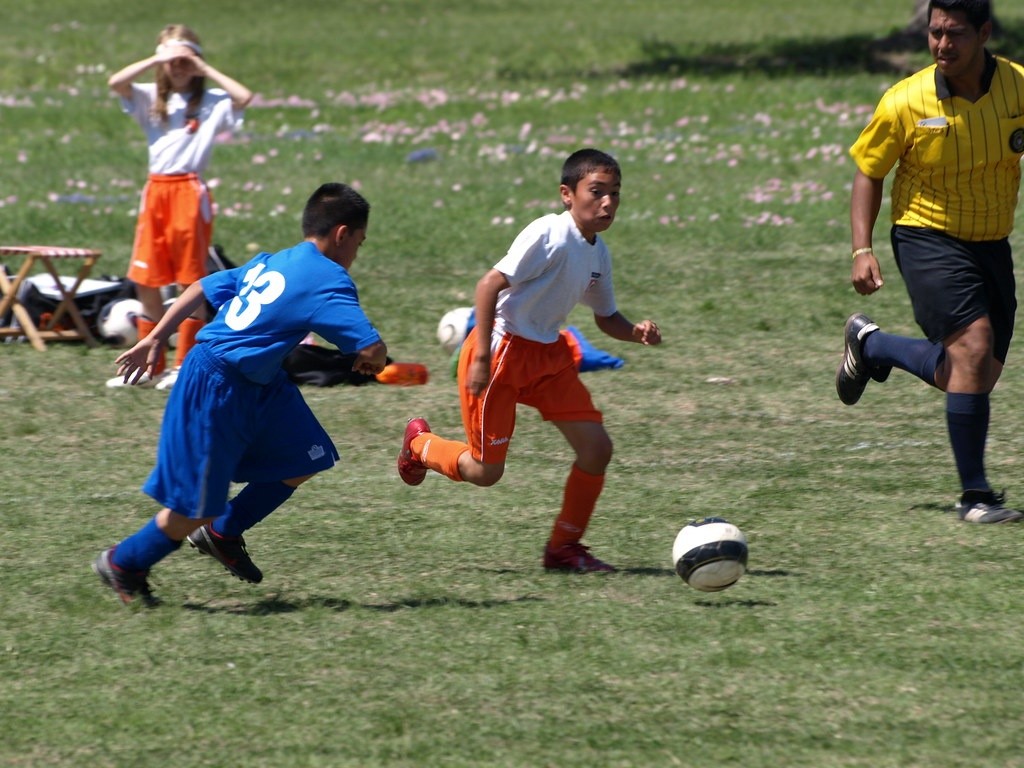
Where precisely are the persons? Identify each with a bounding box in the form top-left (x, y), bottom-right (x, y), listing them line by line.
top-left (836, 0), bottom-right (1024, 525)
top-left (105, 23), bottom-right (255, 390)
top-left (397, 147), bottom-right (663, 575)
top-left (90, 182), bottom-right (388, 612)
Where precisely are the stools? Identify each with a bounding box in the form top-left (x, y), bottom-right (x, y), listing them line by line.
top-left (0, 244), bottom-right (104, 353)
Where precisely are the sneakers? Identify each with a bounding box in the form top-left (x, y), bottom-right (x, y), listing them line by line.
top-left (954, 488), bottom-right (1023, 525)
top-left (398, 417), bottom-right (431, 486)
top-left (544, 541), bottom-right (613, 574)
top-left (836, 313), bottom-right (879, 406)
top-left (105, 368), bottom-right (164, 390)
top-left (90, 545), bottom-right (161, 613)
top-left (154, 366), bottom-right (181, 390)
top-left (185, 522), bottom-right (262, 584)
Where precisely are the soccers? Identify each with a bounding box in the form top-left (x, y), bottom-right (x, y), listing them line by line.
top-left (98, 299), bottom-right (145, 345)
top-left (435, 307), bottom-right (471, 354)
top-left (672, 517), bottom-right (749, 595)
top-left (161, 298), bottom-right (180, 349)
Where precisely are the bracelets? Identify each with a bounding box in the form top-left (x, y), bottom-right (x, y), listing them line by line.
top-left (852, 247), bottom-right (872, 259)
